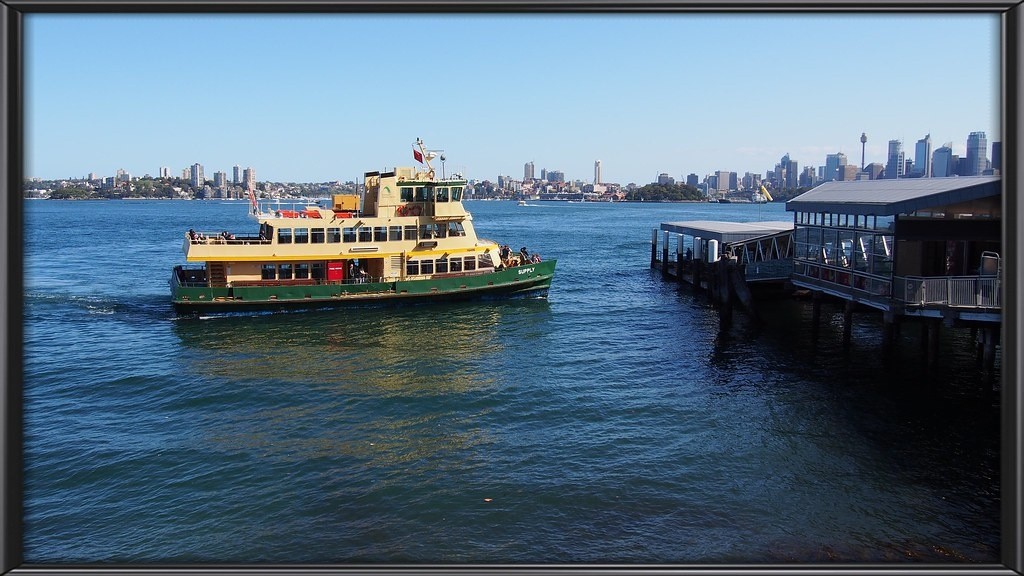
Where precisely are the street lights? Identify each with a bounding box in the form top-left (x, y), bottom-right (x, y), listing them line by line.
top-left (860, 132), bottom-right (868, 172)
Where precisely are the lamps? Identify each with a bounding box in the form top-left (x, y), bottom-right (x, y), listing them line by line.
top-left (420, 241), bottom-right (438, 248)
top-left (350, 248), bottom-right (379, 251)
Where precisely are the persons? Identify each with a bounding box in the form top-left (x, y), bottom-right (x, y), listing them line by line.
top-left (686, 247), bottom-right (692, 260)
top-left (500, 245), bottom-right (542, 267)
top-left (348, 259), bottom-right (368, 285)
top-left (189, 228), bottom-right (267, 244)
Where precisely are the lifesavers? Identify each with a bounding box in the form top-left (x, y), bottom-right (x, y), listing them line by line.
top-left (411, 204), bottom-right (423, 216)
top-left (397, 206), bottom-right (409, 216)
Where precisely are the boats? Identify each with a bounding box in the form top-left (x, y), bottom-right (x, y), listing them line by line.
top-left (167, 138), bottom-right (557, 320)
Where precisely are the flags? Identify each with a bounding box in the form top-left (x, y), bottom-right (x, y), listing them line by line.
top-left (247, 182), bottom-right (257, 208)
top-left (413, 149), bottom-right (423, 164)
